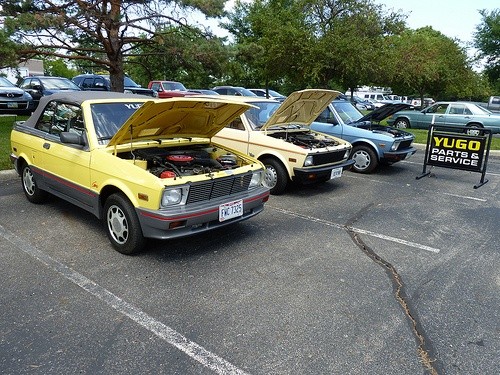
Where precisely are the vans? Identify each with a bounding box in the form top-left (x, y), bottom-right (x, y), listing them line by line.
top-left (363, 93), bottom-right (435, 108)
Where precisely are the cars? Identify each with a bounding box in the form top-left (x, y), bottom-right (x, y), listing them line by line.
top-left (386, 101), bottom-right (500, 137)
top-left (148, 80), bottom-right (288, 104)
top-left (184, 89), bottom-right (356, 195)
top-left (258, 99), bottom-right (417, 174)
top-left (18, 75), bottom-right (84, 102)
top-left (346, 95), bottom-right (386, 110)
top-left (8, 90), bottom-right (270, 255)
top-left (487, 96), bottom-right (500, 111)
top-left (0, 74), bottom-right (33, 116)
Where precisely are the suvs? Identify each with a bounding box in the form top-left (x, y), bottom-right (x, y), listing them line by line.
top-left (71, 73), bottom-right (155, 101)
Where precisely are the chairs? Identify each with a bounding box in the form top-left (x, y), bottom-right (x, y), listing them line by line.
top-left (319, 107), bottom-right (330, 122)
